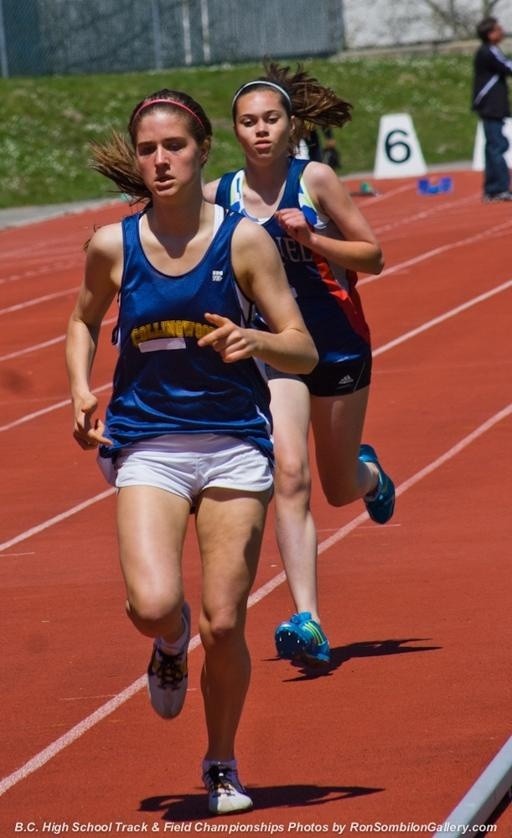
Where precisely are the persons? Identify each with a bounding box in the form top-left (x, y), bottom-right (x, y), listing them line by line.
top-left (471, 18), bottom-right (512, 204)
top-left (65, 88), bottom-right (319, 812)
top-left (303, 123), bottom-right (336, 162)
top-left (203, 60), bottom-right (395, 673)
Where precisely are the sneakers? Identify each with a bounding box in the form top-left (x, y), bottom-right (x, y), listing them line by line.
top-left (274, 612), bottom-right (331, 672)
top-left (482, 189), bottom-right (512, 203)
top-left (357, 443), bottom-right (393, 524)
top-left (202, 764), bottom-right (254, 816)
top-left (146, 602), bottom-right (190, 721)
